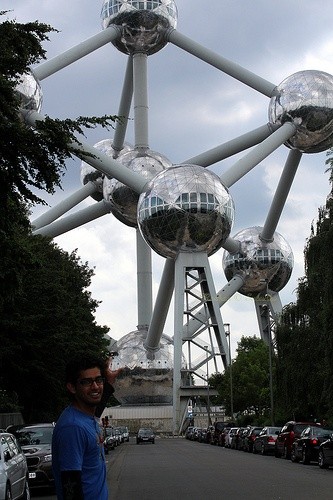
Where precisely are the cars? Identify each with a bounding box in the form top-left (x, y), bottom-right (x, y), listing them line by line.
top-left (184, 421), bottom-right (333, 469)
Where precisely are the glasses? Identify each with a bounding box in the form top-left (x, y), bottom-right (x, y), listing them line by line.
top-left (80, 376), bottom-right (103, 387)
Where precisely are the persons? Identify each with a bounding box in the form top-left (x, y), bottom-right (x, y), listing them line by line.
top-left (51, 361), bottom-right (109, 500)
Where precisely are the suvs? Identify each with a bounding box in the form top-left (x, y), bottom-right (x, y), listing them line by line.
top-left (0, 432), bottom-right (31, 500)
top-left (135, 427), bottom-right (157, 445)
top-left (103, 424), bottom-right (131, 454)
top-left (0, 420), bottom-right (57, 486)
